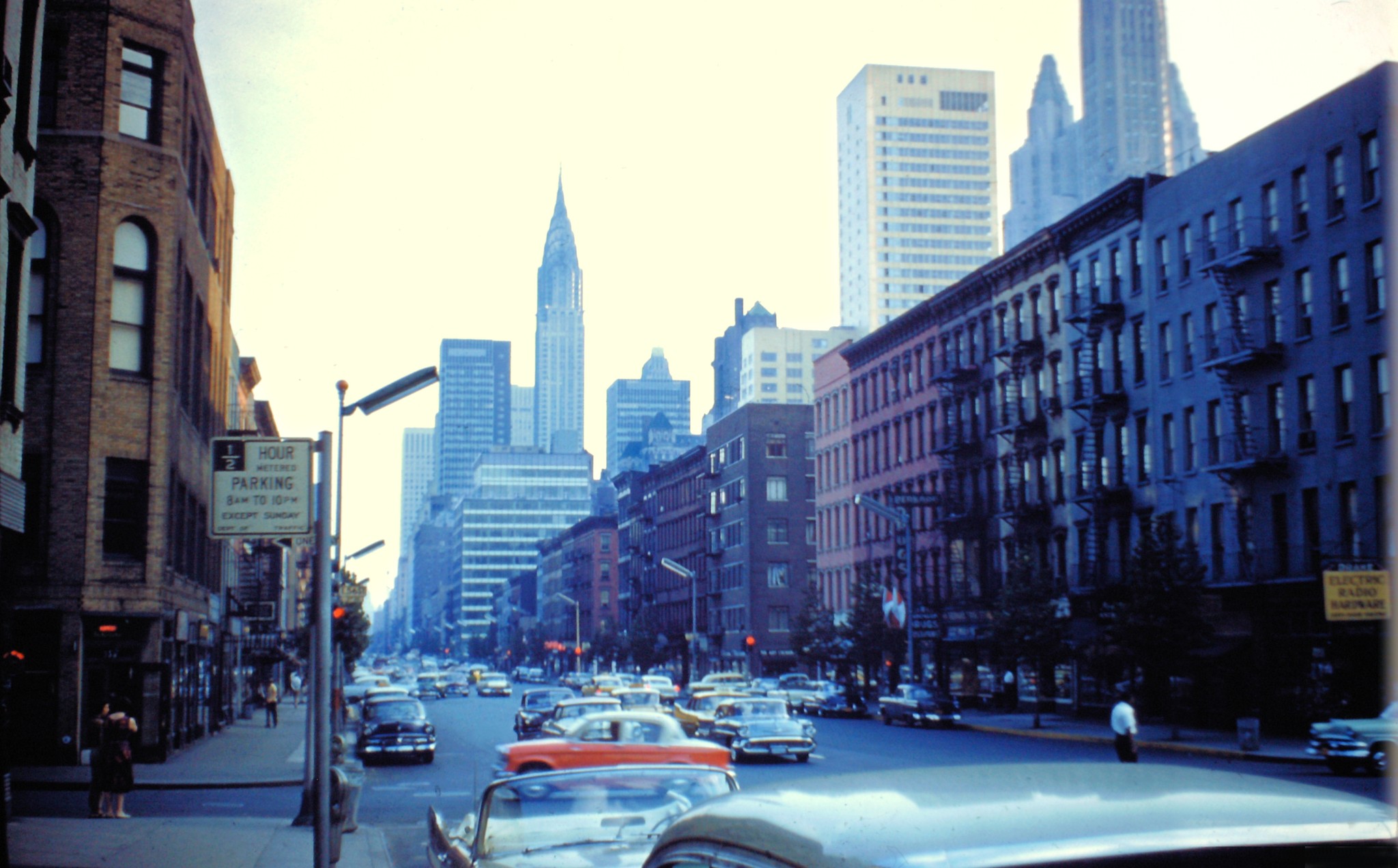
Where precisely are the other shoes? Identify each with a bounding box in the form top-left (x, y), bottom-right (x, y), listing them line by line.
top-left (105, 812), bottom-right (115, 818)
top-left (88, 809), bottom-right (105, 819)
top-left (116, 811), bottom-right (131, 819)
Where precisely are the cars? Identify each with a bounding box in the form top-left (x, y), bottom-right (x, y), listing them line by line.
top-left (639, 760), bottom-right (1398, 868)
top-left (426, 765), bottom-right (742, 868)
top-left (338, 652), bottom-right (512, 767)
top-left (1306, 699), bottom-right (1398, 779)
top-left (512, 664), bottom-right (546, 685)
top-left (489, 668), bottom-right (868, 779)
top-left (878, 683), bottom-right (962, 729)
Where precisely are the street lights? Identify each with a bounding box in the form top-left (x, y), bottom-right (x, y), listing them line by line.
top-left (853, 494), bottom-right (916, 683)
top-left (661, 557), bottom-right (697, 683)
top-left (309, 364), bottom-right (442, 868)
top-left (555, 592), bottom-right (581, 673)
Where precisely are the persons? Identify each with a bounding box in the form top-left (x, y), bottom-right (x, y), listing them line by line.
top-left (290, 672), bottom-right (301, 708)
top-left (88, 700), bottom-right (137, 819)
top-left (1109, 692), bottom-right (1139, 763)
top-left (264, 678), bottom-right (277, 728)
